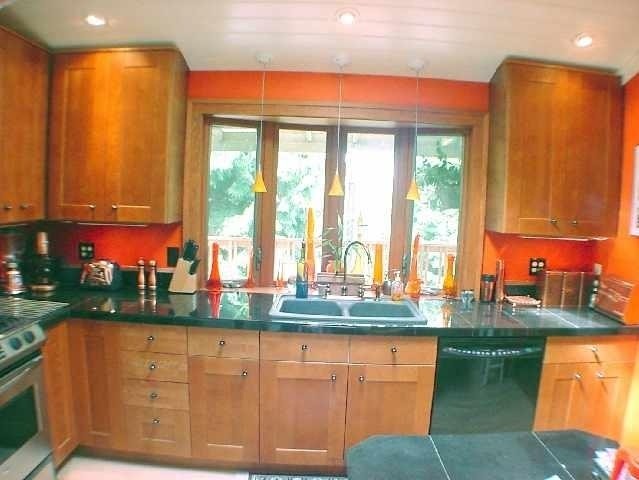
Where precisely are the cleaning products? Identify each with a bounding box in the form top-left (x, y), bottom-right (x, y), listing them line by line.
top-left (391, 271), bottom-right (403, 302)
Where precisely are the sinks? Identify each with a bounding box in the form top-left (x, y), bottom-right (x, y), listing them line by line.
top-left (348, 299), bottom-right (428, 325)
top-left (268, 293), bottom-right (348, 322)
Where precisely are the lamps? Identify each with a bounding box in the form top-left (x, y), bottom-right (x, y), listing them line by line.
top-left (405, 62), bottom-right (424, 201)
top-left (251, 53), bottom-right (271, 193)
top-left (327, 55), bottom-right (353, 195)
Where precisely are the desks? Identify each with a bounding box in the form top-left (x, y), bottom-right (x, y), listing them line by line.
top-left (343, 429), bottom-right (622, 480)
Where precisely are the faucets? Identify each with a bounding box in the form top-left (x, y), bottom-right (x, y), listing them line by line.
top-left (340, 240), bottom-right (373, 297)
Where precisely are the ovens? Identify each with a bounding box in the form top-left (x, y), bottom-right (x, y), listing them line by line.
top-left (0, 355), bottom-right (56, 480)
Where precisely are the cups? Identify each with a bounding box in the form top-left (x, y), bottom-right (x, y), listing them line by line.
top-left (481, 274), bottom-right (496, 302)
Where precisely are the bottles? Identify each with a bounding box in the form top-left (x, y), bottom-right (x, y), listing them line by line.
top-left (296, 239), bottom-right (309, 298)
top-left (4, 255), bottom-right (27, 299)
top-left (31, 232), bottom-right (55, 292)
top-left (589, 278), bottom-right (599, 311)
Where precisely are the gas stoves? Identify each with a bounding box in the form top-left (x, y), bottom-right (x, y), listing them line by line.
top-left (0, 318), bottom-right (46, 377)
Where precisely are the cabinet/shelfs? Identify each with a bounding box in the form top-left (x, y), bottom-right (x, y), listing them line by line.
top-left (188, 325), bottom-right (261, 473)
top-left (532, 334), bottom-right (639, 440)
top-left (47, 47), bottom-right (190, 225)
top-left (259, 329), bottom-right (345, 475)
top-left (67, 318), bottom-right (191, 468)
top-left (344, 336), bottom-right (438, 468)
top-left (1, 24), bottom-right (50, 227)
top-left (42, 321), bottom-right (77, 470)
top-left (488, 59), bottom-right (622, 239)
